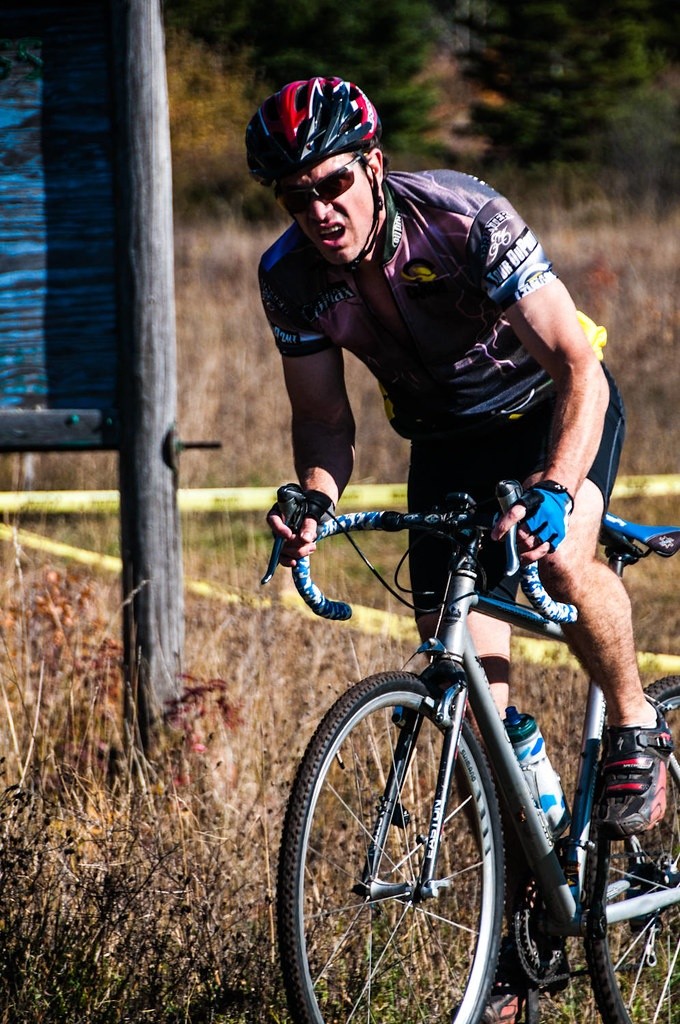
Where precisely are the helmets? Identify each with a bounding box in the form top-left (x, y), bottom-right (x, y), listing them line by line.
top-left (245, 76), bottom-right (382, 186)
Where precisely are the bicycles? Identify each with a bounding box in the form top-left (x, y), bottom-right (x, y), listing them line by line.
top-left (260, 477), bottom-right (680, 1024)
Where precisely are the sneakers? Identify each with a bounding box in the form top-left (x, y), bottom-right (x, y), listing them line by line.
top-left (594, 698), bottom-right (674, 839)
top-left (450, 936), bottom-right (569, 1024)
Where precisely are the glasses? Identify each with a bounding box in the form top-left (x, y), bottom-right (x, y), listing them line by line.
top-left (280, 153), bottom-right (367, 213)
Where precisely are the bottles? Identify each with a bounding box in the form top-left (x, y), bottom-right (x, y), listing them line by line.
top-left (501, 706), bottom-right (572, 843)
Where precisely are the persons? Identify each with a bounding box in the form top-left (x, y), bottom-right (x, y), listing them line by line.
top-left (243, 75), bottom-right (673, 1023)
